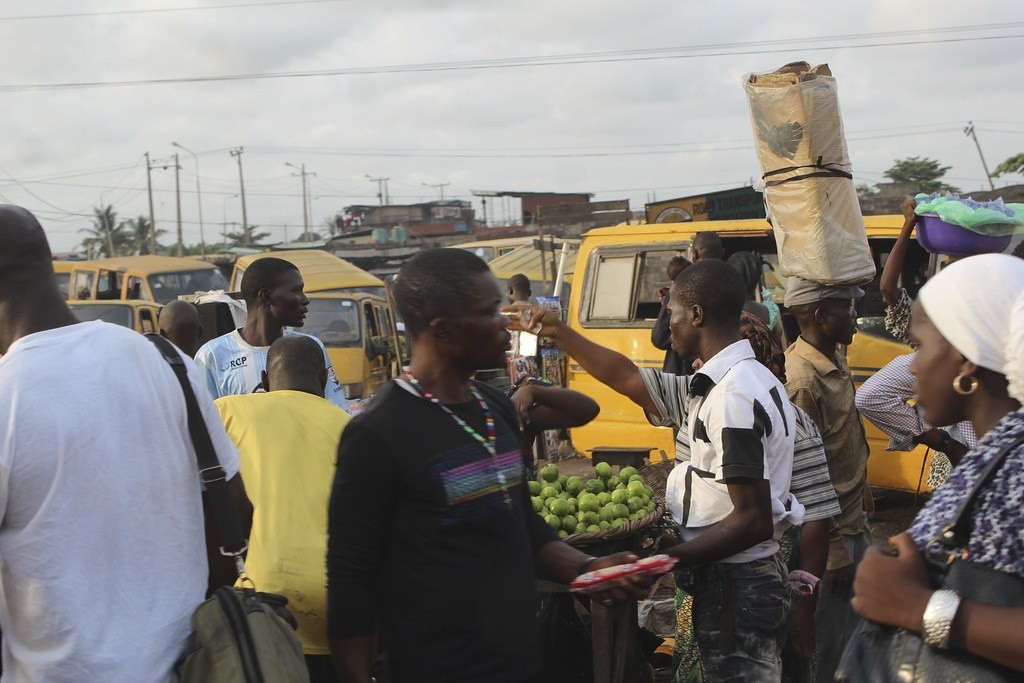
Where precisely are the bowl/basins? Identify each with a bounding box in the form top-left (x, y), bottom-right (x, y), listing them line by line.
top-left (915, 213), bottom-right (1024, 256)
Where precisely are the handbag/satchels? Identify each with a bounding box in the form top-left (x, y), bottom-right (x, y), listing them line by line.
top-left (832, 428), bottom-right (1024, 683)
top-left (173, 574), bottom-right (314, 683)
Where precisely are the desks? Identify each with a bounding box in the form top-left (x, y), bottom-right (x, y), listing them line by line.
top-left (537, 524), bottom-right (668, 683)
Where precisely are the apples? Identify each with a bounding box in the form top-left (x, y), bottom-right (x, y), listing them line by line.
top-left (530, 462), bottom-right (658, 538)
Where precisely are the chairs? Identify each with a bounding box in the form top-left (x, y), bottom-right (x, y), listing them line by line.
top-left (328, 320), bottom-right (350, 332)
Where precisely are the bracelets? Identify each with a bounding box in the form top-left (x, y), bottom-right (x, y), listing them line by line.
top-left (921, 589), bottom-right (963, 651)
top-left (935, 430), bottom-right (950, 453)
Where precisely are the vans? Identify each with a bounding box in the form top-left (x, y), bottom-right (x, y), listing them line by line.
top-left (565, 214), bottom-right (939, 496)
top-left (447, 234), bottom-right (554, 264)
top-left (285, 292), bottom-right (400, 400)
top-left (64, 299), bottom-right (163, 335)
top-left (69, 256), bottom-right (230, 305)
top-left (487, 239), bottom-right (584, 320)
top-left (51, 260), bottom-right (87, 300)
top-left (230, 249), bottom-right (388, 300)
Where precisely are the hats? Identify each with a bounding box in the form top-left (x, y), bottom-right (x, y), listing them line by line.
top-left (784, 274), bottom-right (866, 308)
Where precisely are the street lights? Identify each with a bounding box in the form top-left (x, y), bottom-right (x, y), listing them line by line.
top-left (171, 142), bottom-right (207, 261)
top-left (222, 193), bottom-right (238, 251)
top-left (963, 120), bottom-right (998, 200)
top-left (285, 162), bottom-right (315, 241)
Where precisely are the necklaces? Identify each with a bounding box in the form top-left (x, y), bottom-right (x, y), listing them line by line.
top-left (401, 363), bottom-right (513, 509)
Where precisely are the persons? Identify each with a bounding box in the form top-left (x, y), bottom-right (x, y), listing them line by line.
top-left (159, 193), bottom-right (1023, 683)
top-left (0, 202), bottom-right (254, 682)
